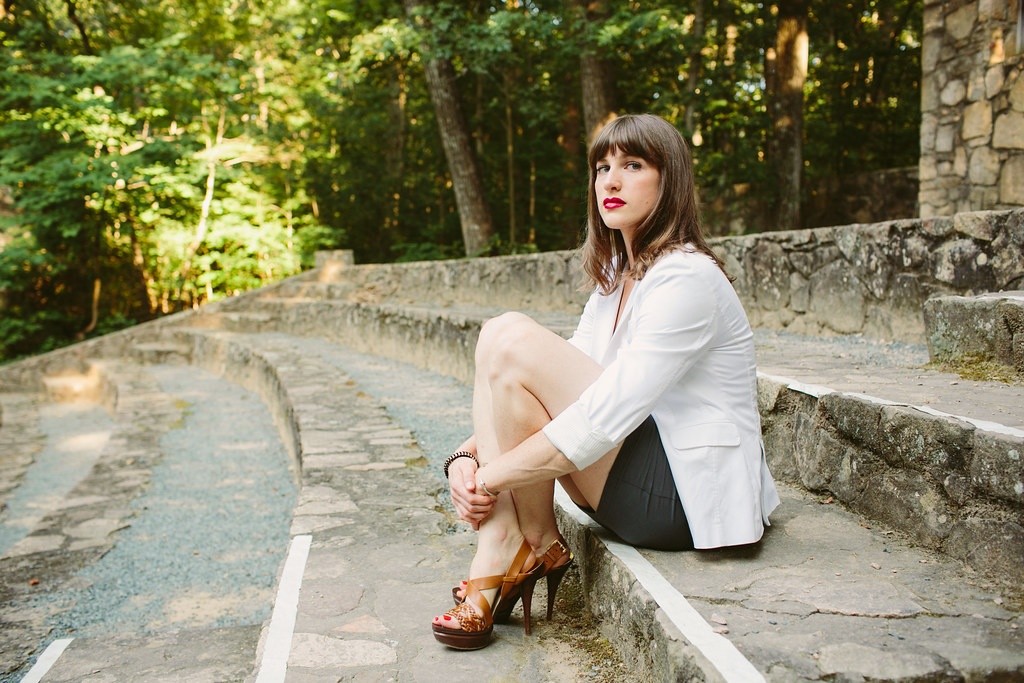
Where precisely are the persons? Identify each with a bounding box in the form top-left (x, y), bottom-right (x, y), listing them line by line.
top-left (431, 115), bottom-right (782, 650)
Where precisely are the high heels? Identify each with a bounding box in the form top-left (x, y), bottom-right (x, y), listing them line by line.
top-left (453, 536), bottom-right (574, 621)
top-left (433, 536), bottom-right (546, 653)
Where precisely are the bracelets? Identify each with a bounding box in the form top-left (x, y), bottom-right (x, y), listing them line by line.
top-left (479, 462), bottom-right (500, 497)
top-left (443, 451), bottom-right (479, 478)
